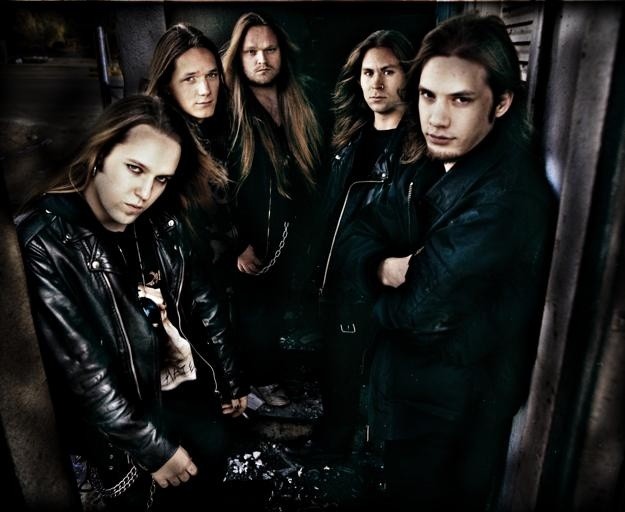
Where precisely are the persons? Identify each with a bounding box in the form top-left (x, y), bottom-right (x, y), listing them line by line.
top-left (314, 28), bottom-right (419, 303)
top-left (12, 94), bottom-right (250, 512)
top-left (146, 22), bottom-right (245, 323)
top-left (331, 12), bottom-right (561, 511)
top-left (219, 12), bottom-right (328, 322)
